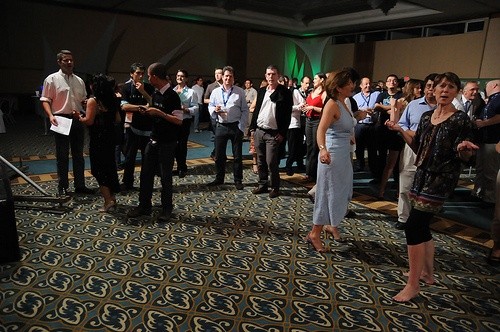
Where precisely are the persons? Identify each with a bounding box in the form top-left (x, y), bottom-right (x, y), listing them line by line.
top-left (78, 74), bottom-right (121, 212)
top-left (82, 54), bottom-right (500, 268)
top-left (384, 72), bottom-right (481, 302)
top-left (307, 68), bottom-right (356, 252)
top-left (127, 62), bottom-right (183, 221)
top-left (172, 69), bottom-right (199, 178)
top-left (40, 50), bottom-right (97, 196)
top-left (206, 66), bottom-right (249, 190)
top-left (247, 66), bottom-right (293, 198)
top-left (301, 73), bottom-right (328, 184)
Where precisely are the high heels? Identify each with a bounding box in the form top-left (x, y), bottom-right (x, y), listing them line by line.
top-left (323, 227), bottom-right (346, 242)
top-left (306, 232), bottom-right (330, 253)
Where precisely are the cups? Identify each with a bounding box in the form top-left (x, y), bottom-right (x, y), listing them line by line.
top-left (36, 90), bottom-right (39, 96)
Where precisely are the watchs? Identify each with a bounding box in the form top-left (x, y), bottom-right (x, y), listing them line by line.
top-left (138, 104), bottom-right (143, 112)
top-left (319, 145), bottom-right (325, 150)
top-left (160, 113), bottom-right (167, 119)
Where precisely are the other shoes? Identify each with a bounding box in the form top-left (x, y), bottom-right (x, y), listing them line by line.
top-left (306, 193), bottom-right (315, 205)
top-left (252, 164), bottom-right (259, 174)
top-left (210, 154), bottom-right (216, 161)
top-left (234, 181), bottom-right (244, 190)
top-left (251, 186), bottom-right (269, 194)
top-left (285, 165), bottom-right (295, 176)
top-left (121, 182), bottom-right (134, 195)
top-left (487, 248), bottom-right (500, 266)
top-left (104, 200), bottom-right (117, 213)
top-left (210, 135), bottom-right (215, 141)
top-left (293, 166), bottom-right (306, 172)
top-left (75, 186), bottom-right (96, 195)
top-left (395, 221), bottom-right (407, 229)
top-left (58, 187), bottom-right (68, 197)
top-left (157, 212), bottom-right (173, 222)
top-left (207, 177), bottom-right (224, 186)
top-left (113, 181), bottom-right (120, 193)
top-left (300, 176), bottom-right (314, 183)
top-left (476, 186), bottom-right (485, 197)
top-left (178, 164), bottom-right (187, 178)
top-left (127, 205), bottom-right (153, 218)
top-left (269, 189), bottom-right (282, 198)
